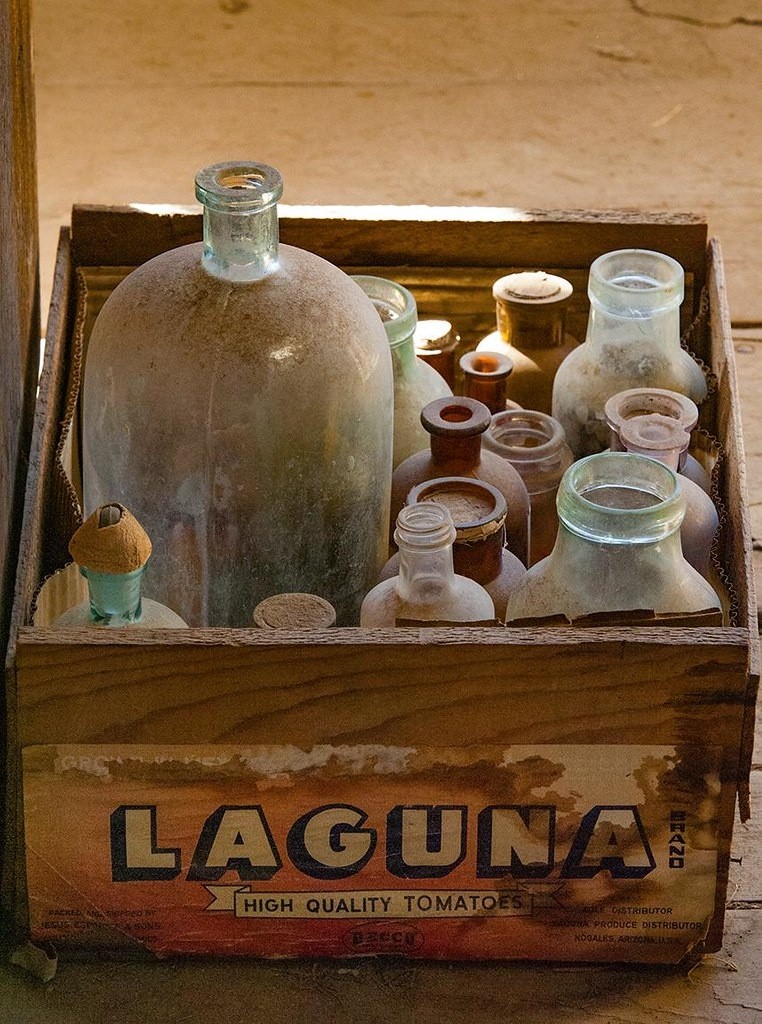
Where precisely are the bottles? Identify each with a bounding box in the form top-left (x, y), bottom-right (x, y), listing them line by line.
top-left (48, 159), bottom-right (726, 633)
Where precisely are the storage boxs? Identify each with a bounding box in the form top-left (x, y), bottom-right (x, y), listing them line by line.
top-left (0, 202), bottom-right (762, 963)
